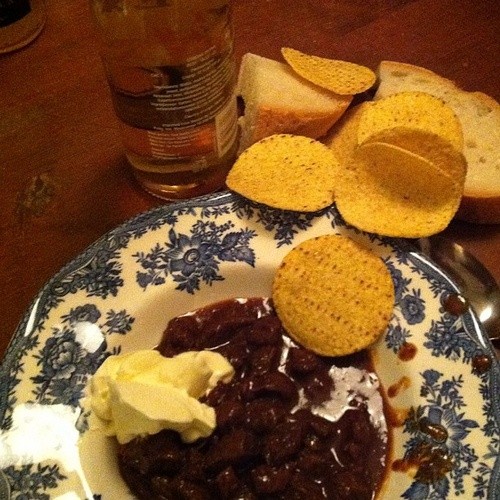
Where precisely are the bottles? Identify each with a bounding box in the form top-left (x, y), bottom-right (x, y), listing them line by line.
top-left (90, 0), bottom-right (236, 202)
top-left (1, 0), bottom-right (46, 53)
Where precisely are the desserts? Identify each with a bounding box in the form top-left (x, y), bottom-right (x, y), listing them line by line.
top-left (85, 349), bottom-right (235, 443)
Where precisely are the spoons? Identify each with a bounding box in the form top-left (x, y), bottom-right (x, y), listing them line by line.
top-left (406, 231), bottom-right (498, 339)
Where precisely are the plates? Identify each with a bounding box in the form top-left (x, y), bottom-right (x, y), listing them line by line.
top-left (1, 187), bottom-right (499, 500)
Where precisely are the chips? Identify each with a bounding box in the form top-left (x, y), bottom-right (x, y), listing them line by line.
top-left (225, 47), bottom-right (500, 356)
